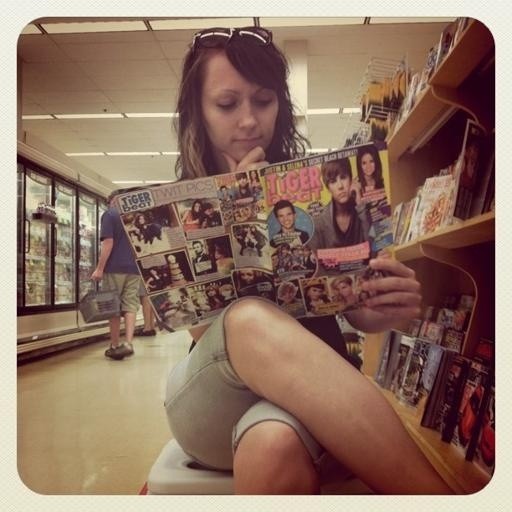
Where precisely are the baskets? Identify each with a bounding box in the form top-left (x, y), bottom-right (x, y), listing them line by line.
top-left (79, 289), bottom-right (121, 323)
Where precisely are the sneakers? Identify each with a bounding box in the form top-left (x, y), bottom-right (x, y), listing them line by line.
top-left (134, 328), bottom-right (157, 336)
top-left (105, 341), bottom-right (134, 361)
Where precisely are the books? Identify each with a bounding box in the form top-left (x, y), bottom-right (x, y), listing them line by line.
top-left (393, 121), bottom-right (495, 243)
top-left (372, 297), bottom-right (495, 475)
top-left (114, 141), bottom-right (397, 332)
top-left (347, 17), bottom-right (472, 143)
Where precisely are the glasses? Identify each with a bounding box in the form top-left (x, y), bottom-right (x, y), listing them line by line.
top-left (190, 26), bottom-right (275, 57)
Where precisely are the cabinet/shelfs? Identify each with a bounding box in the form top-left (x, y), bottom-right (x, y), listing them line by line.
top-left (348, 17), bottom-right (495, 494)
top-left (17, 155), bottom-right (109, 316)
top-left (342, 54), bottom-right (412, 155)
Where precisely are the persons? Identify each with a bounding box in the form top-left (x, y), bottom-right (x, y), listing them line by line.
top-left (271, 200), bottom-right (309, 247)
top-left (271, 243), bottom-right (317, 307)
top-left (310, 157), bottom-right (378, 275)
top-left (146, 254), bottom-right (191, 293)
top-left (333, 275), bottom-right (374, 307)
top-left (304, 278), bottom-right (331, 313)
top-left (91, 187), bottom-right (140, 361)
top-left (128, 207), bottom-right (172, 253)
top-left (162, 24), bottom-right (464, 494)
top-left (162, 284), bottom-right (235, 326)
top-left (191, 241), bottom-right (234, 275)
top-left (218, 172), bottom-right (274, 300)
top-left (181, 199), bottom-right (223, 234)
top-left (134, 275), bottom-right (159, 338)
top-left (355, 149), bottom-right (390, 232)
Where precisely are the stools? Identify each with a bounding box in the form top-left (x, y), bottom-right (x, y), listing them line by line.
top-left (148, 435), bottom-right (239, 493)
